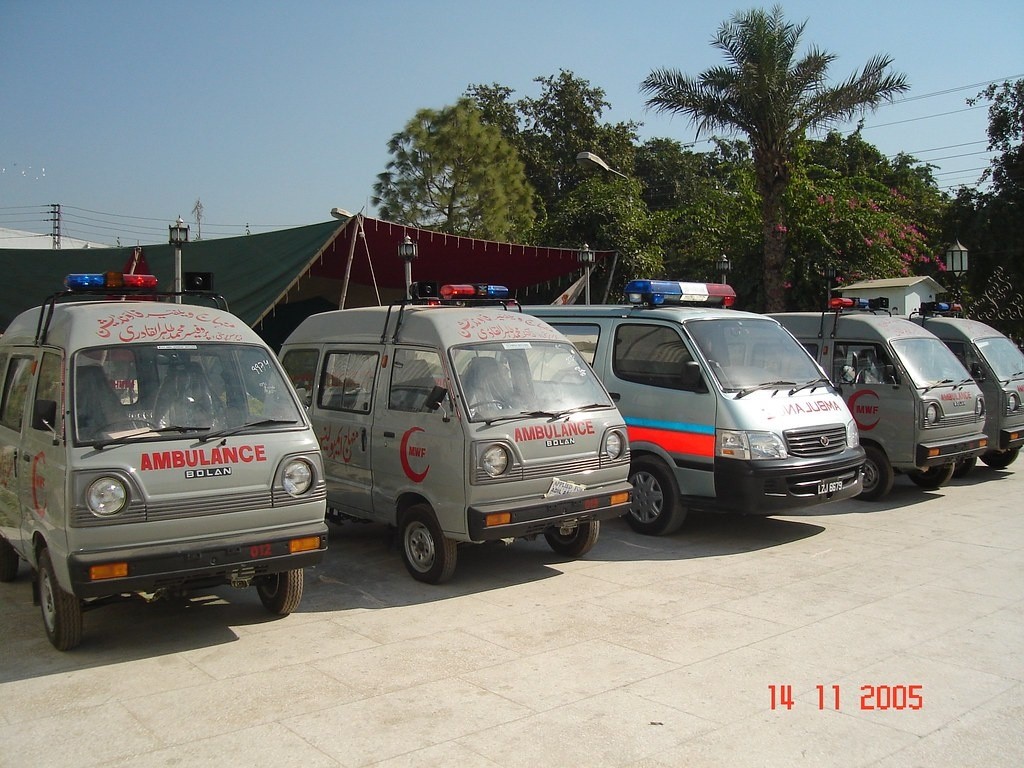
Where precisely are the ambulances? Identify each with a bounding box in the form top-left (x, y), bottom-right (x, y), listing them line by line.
top-left (0, 273), bottom-right (328, 649)
top-left (762, 298), bottom-right (989, 501)
top-left (278, 283), bottom-right (633, 584)
top-left (501, 279), bottom-right (866, 535)
top-left (867, 303), bottom-right (1024, 467)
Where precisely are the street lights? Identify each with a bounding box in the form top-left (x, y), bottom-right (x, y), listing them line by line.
top-left (946, 239), bottom-right (969, 294)
top-left (577, 243), bottom-right (595, 305)
top-left (576, 152), bottom-right (633, 212)
top-left (714, 253), bottom-right (731, 309)
top-left (168, 215), bottom-right (189, 303)
top-left (398, 235), bottom-right (419, 300)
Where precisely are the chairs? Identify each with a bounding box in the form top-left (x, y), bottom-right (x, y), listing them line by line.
top-left (153, 363), bottom-right (230, 433)
top-left (390, 359), bottom-right (451, 414)
top-left (461, 357), bottom-right (513, 412)
top-left (77, 366), bottom-right (136, 429)
